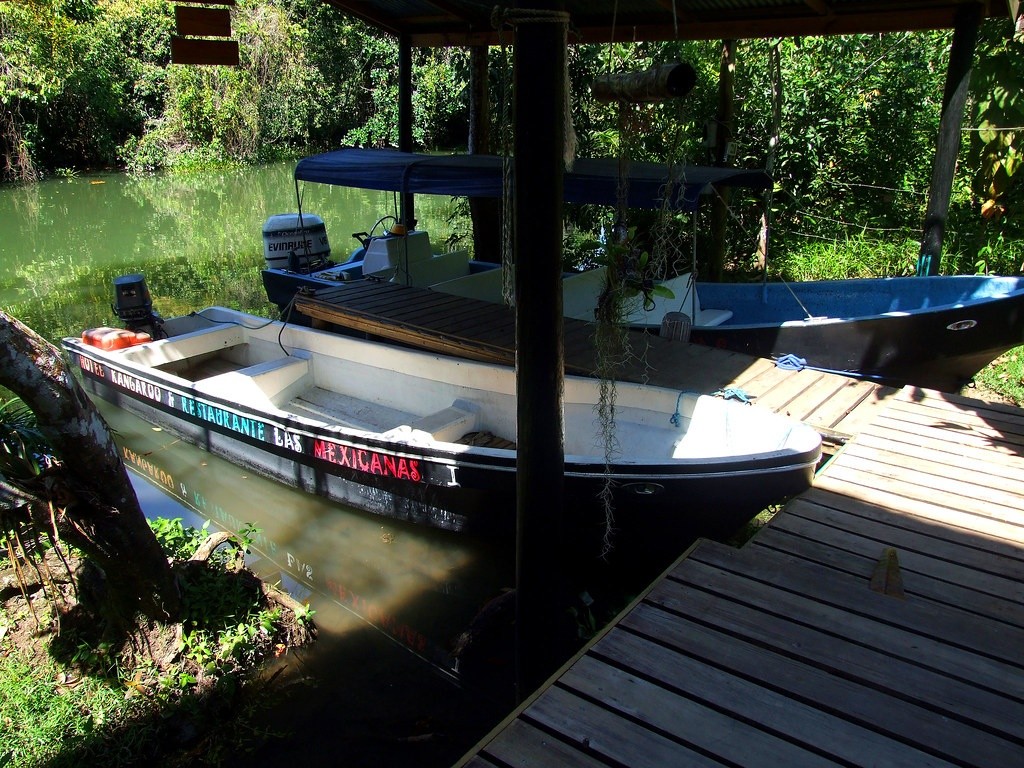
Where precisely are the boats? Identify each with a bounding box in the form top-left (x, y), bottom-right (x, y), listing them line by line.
top-left (261, 151), bottom-right (1024, 396)
top-left (60, 275), bottom-right (824, 563)
